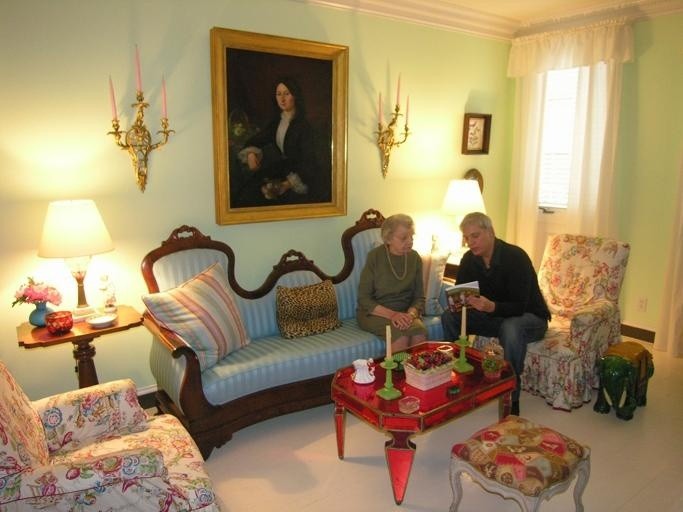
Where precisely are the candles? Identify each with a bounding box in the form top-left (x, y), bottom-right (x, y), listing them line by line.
top-left (397, 73), bottom-right (400, 106)
top-left (378, 92), bottom-right (382, 122)
top-left (108, 76), bottom-right (118, 121)
top-left (406, 96), bottom-right (409, 124)
top-left (386, 324), bottom-right (392, 362)
top-left (134, 45), bottom-right (143, 94)
top-left (461, 307), bottom-right (467, 339)
top-left (162, 78), bottom-right (168, 117)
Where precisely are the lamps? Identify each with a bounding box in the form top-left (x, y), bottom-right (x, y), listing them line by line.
top-left (38, 199), bottom-right (114, 322)
top-left (443, 180), bottom-right (485, 217)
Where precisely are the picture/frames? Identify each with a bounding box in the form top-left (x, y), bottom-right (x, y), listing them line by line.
top-left (463, 168), bottom-right (484, 194)
top-left (460, 113), bottom-right (492, 155)
top-left (210, 27), bottom-right (348, 226)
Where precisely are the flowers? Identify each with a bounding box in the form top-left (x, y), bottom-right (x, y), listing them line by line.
top-left (408, 351), bottom-right (454, 369)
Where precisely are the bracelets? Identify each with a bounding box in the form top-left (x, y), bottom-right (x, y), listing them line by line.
top-left (409, 311), bottom-right (416, 319)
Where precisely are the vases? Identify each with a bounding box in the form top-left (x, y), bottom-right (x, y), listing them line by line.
top-left (400, 359), bottom-right (452, 391)
top-left (30, 301), bottom-right (54, 326)
top-left (12, 277), bottom-right (64, 309)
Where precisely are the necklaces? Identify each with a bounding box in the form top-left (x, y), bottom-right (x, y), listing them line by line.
top-left (385, 242), bottom-right (409, 282)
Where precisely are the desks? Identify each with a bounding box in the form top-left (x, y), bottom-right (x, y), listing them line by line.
top-left (15, 302), bottom-right (144, 390)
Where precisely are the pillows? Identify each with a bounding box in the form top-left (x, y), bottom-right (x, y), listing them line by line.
top-left (141, 262), bottom-right (251, 374)
top-left (275, 281), bottom-right (345, 340)
top-left (416, 250), bottom-right (455, 316)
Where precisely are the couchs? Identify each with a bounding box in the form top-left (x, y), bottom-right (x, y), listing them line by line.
top-left (139, 208), bottom-right (455, 461)
top-left (473, 233), bottom-right (631, 412)
top-left (1, 361), bottom-right (220, 512)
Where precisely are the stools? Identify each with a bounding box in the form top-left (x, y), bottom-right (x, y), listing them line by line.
top-left (449, 415), bottom-right (592, 512)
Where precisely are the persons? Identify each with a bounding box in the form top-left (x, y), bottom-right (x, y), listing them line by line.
top-left (436, 210), bottom-right (555, 421)
top-left (352, 212), bottom-right (432, 356)
top-left (232, 70), bottom-right (322, 205)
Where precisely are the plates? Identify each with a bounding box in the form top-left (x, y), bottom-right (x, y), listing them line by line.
top-left (84, 313), bottom-right (119, 329)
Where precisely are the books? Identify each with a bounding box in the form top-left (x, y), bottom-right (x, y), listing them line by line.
top-left (443, 280), bottom-right (482, 316)
